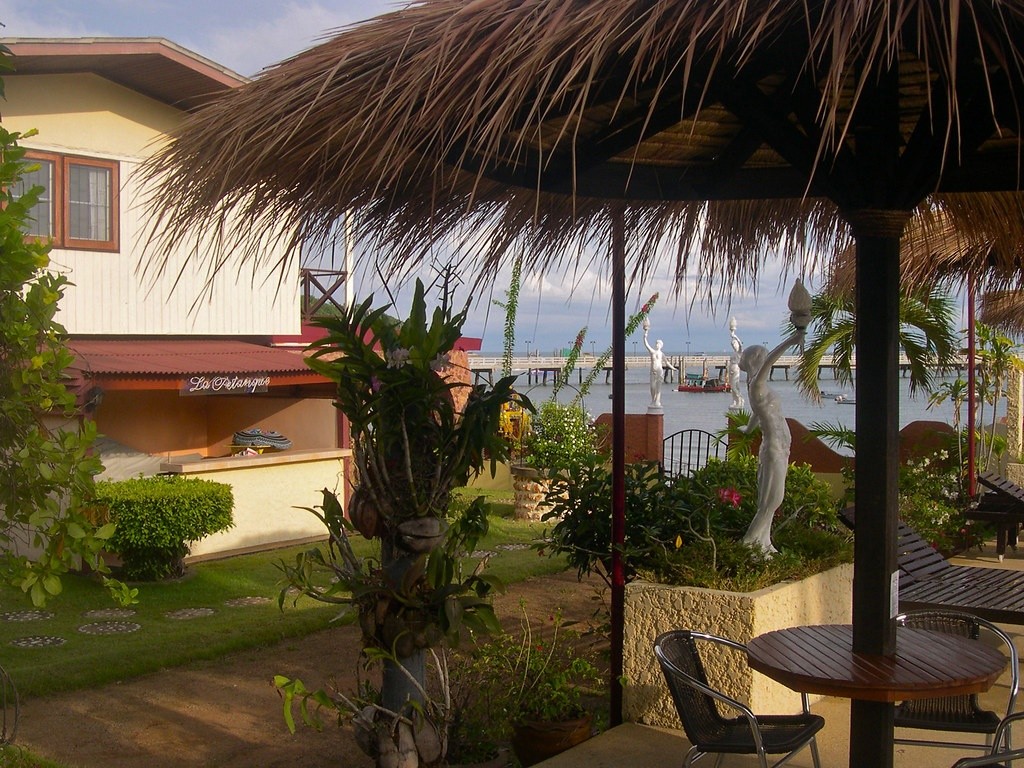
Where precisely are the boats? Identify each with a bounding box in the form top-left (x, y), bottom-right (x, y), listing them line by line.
top-left (677, 380), bottom-right (733, 394)
top-left (820, 391), bottom-right (856, 404)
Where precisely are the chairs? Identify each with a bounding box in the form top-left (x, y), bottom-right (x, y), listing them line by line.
top-left (662, 468), bottom-right (1024, 768)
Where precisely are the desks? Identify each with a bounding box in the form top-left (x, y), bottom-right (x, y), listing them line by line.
top-left (223, 444), bottom-right (272, 455)
top-left (747, 625), bottom-right (1009, 768)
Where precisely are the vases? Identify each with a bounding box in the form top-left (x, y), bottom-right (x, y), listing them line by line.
top-left (510, 706), bottom-right (593, 768)
top-left (508, 464), bottom-right (597, 523)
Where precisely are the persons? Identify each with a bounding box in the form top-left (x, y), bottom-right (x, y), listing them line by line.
top-left (644, 333), bottom-right (678, 406)
top-left (728, 334), bottom-right (744, 407)
top-left (737, 330), bottom-right (805, 559)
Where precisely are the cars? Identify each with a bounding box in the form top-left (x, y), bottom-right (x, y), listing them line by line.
top-left (696, 351), bottom-right (706, 356)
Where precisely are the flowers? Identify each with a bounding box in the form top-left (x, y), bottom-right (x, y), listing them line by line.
top-left (469, 594), bottom-right (629, 728)
top-left (521, 292), bottom-right (661, 464)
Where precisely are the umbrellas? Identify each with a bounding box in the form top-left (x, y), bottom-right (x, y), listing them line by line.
top-left (134, 1), bottom-right (1024, 651)
top-left (827, 206), bottom-right (1024, 495)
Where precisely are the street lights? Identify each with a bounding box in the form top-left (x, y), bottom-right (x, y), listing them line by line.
top-left (686, 341), bottom-right (691, 357)
top-left (568, 341), bottom-right (574, 352)
top-left (525, 341), bottom-right (531, 358)
top-left (632, 341), bottom-right (637, 357)
top-left (590, 341), bottom-right (596, 358)
